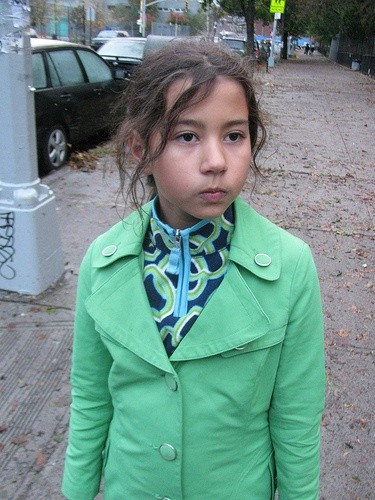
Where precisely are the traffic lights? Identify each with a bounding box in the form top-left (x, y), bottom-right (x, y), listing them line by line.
top-left (185, 0), bottom-right (188, 9)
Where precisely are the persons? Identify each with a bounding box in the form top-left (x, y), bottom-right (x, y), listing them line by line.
top-left (61, 36), bottom-right (326, 500)
top-left (311, 43), bottom-right (315, 54)
top-left (305, 44), bottom-right (310, 55)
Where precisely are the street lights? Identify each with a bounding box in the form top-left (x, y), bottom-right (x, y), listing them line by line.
top-left (80, 0), bottom-right (86, 45)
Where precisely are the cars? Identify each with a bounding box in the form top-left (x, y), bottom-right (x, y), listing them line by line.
top-left (30, 37), bottom-right (131, 177)
top-left (216, 37), bottom-right (271, 67)
top-left (90, 28), bottom-right (130, 50)
top-left (96, 36), bottom-right (146, 71)
